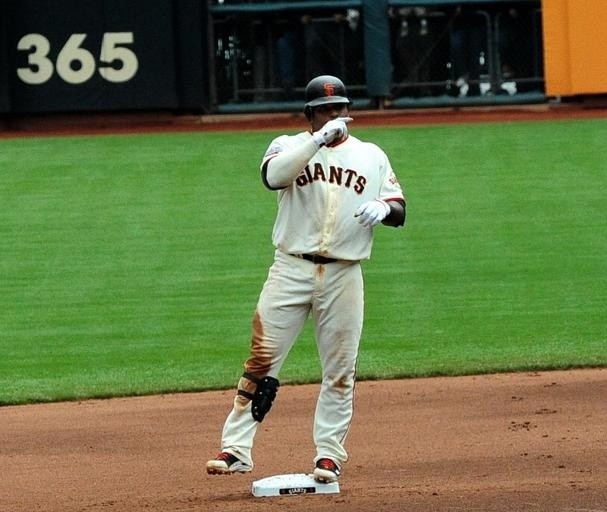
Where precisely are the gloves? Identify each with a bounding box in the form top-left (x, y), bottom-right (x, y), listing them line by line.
top-left (355, 197), bottom-right (391, 227)
top-left (313, 117), bottom-right (353, 144)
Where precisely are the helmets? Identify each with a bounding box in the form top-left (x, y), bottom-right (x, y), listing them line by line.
top-left (304, 75), bottom-right (350, 109)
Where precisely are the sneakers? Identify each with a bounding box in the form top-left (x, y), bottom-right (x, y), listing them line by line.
top-left (313, 458), bottom-right (340, 482)
top-left (206, 452), bottom-right (252, 475)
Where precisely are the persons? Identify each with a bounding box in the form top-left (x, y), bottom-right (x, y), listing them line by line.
top-left (203, 73), bottom-right (408, 485)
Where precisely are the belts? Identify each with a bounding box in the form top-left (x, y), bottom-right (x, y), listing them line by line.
top-left (290, 254), bottom-right (337, 263)
